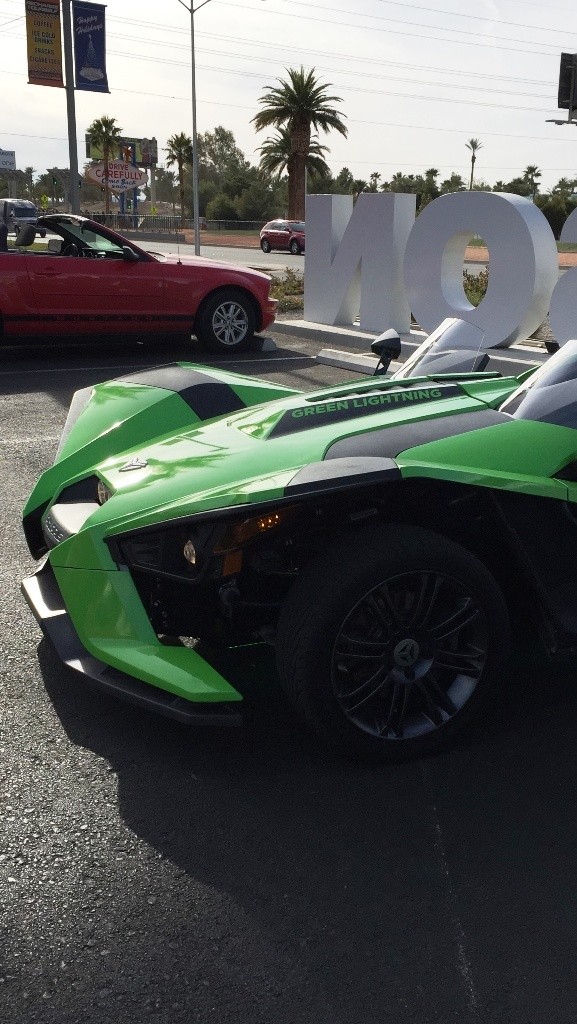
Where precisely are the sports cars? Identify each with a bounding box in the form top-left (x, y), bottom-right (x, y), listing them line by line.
top-left (19, 315), bottom-right (577, 769)
top-left (0, 213), bottom-right (280, 355)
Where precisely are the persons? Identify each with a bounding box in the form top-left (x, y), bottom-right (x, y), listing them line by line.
top-left (35, 207), bottom-right (132, 229)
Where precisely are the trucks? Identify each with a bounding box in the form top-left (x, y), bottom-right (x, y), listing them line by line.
top-left (0, 198), bottom-right (47, 238)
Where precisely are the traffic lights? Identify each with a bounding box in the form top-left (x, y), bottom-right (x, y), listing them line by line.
top-left (77, 180), bottom-right (81, 188)
top-left (101, 186), bottom-right (105, 192)
top-left (127, 200), bottom-right (131, 209)
top-left (53, 179), bottom-right (56, 185)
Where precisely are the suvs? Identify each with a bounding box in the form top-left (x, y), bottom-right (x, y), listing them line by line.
top-left (259, 218), bottom-right (306, 255)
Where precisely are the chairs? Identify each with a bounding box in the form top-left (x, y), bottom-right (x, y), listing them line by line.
top-left (15, 223), bottom-right (37, 254)
